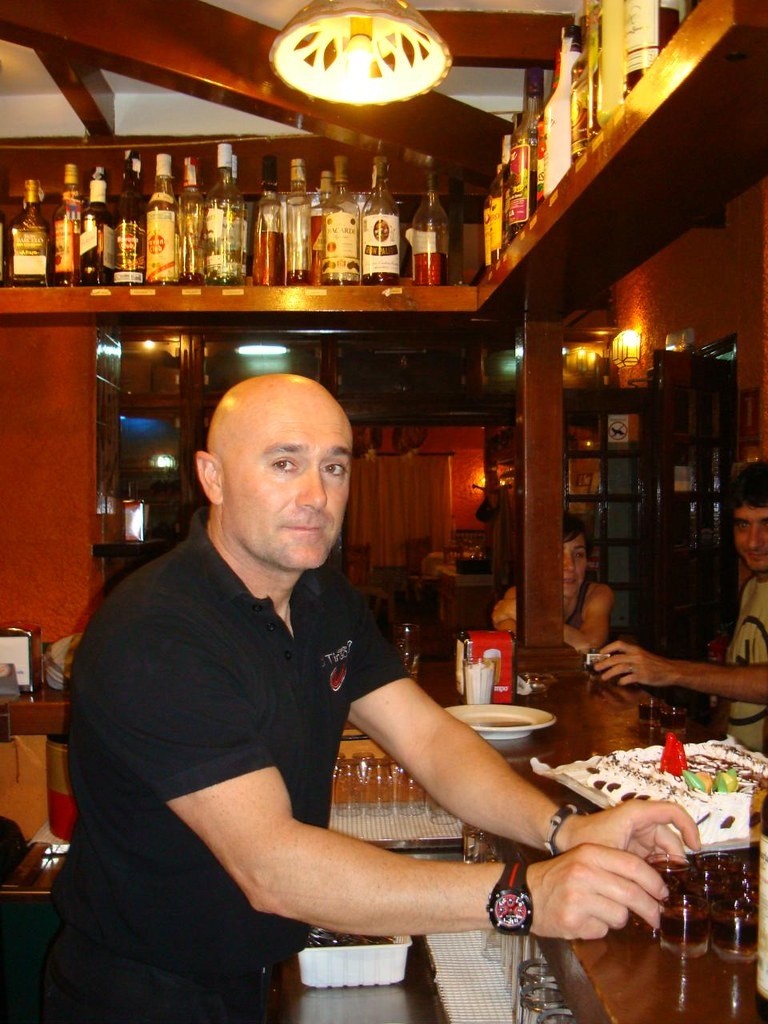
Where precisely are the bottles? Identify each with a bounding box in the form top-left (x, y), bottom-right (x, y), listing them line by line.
top-left (0, 143), bottom-right (452, 288)
top-left (484, 0), bottom-right (693, 268)
top-left (754, 794), bottom-right (768, 1022)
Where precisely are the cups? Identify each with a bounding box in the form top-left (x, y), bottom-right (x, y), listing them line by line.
top-left (636, 696), bottom-right (688, 738)
top-left (392, 622), bottom-right (421, 677)
top-left (460, 826), bottom-right (578, 1024)
top-left (591, 657), bottom-right (611, 681)
top-left (644, 849), bottom-right (760, 964)
top-left (483, 648), bottom-right (501, 685)
top-left (462, 658), bottom-right (496, 705)
top-left (330, 750), bottom-right (459, 822)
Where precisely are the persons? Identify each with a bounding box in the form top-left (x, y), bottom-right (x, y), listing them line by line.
top-left (45, 375), bottom-right (703, 1024)
top-left (490, 516), bottom-right (615, 653)
top-left (594, 463), bottom-right (768, 762)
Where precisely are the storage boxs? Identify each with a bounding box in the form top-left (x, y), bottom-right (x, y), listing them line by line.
top-left (298, 931), bottom-right (414, 988)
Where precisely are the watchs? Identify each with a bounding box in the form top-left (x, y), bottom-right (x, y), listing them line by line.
top-left (487, 859), bottom-right (534, 940)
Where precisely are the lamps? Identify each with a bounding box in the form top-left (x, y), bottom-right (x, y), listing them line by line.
top-left (612, 330), bottom-right (642, 368)
top-left (268, 0), bottom-right (452, 108)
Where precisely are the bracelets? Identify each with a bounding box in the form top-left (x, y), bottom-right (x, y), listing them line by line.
top-left (543, 804), bottom-right (588, 857)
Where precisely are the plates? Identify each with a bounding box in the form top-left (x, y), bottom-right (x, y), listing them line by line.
top-left (445, 703), bottom-right (557, 740)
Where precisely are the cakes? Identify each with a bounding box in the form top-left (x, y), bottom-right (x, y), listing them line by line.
top-left (586, 731), bottom-right (768, 847)
